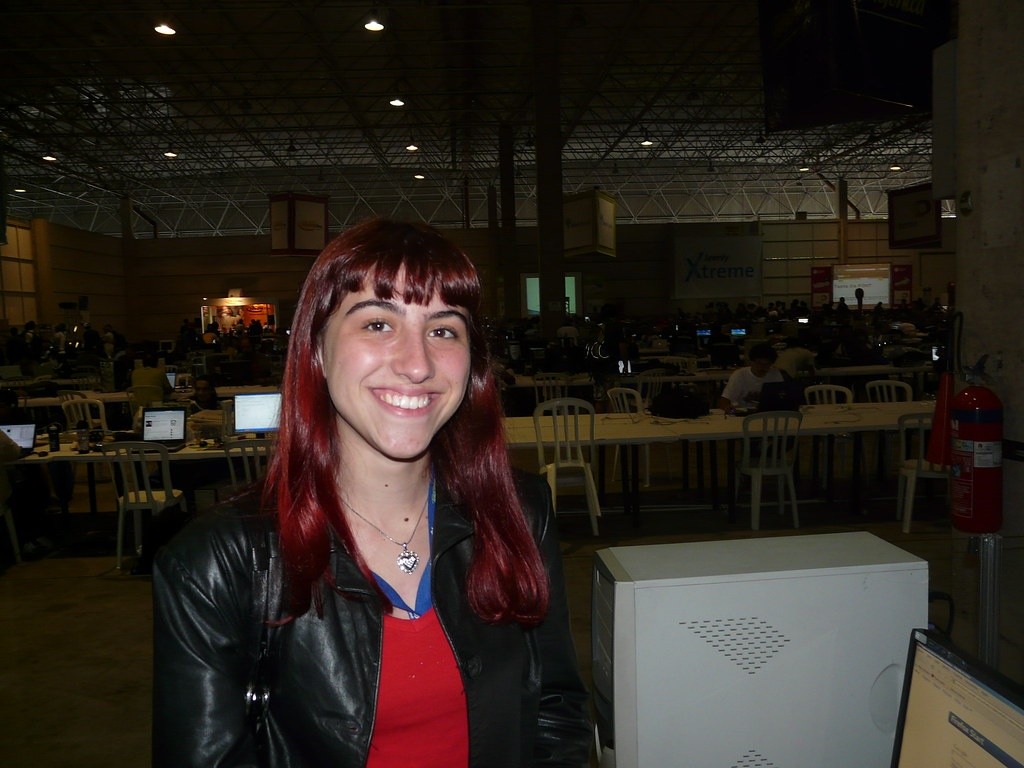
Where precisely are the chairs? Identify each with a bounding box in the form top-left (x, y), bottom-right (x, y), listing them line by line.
top-left (899, 412), bottom-right (950, 534)
top-left (224, 439), bottom-right (277, 493)
top-left (605, 386), bottom-right (648, 487)
top-left (55, 385), bottom-right (110, 429)
top-left (864, 380), bottom-right (913, 477)
top-left (534, 397), bottom-right (601, 537)
top-left (532, 371), bottom-right (572, 415)
top-left (101, 443), bottom-right (185, 570)
top-left (733, 409), bottom-right (802, 532)
top-left (805, 384), bottom-right (866, 483)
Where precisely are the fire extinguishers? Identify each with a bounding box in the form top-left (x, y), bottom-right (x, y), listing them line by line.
top-left (926, 354), bottom-right (1003, 533)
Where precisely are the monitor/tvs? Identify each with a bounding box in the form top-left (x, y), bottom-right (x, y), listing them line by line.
top-left (234, 392), bottom-right (283, 439)
top-left (160, 340), bottom-right (175, 352)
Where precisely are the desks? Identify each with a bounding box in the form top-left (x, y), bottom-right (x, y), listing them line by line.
top-left (0, 431), bottom-right (281, 541)
top-left (6, 331), bottom-right (940, 411)
top-left (502, 398), bottom-right (938, 527)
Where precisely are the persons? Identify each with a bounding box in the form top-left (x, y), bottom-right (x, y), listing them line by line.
top-left (0, 433), bottom-right (40, 555)
top-left (1, 314), bottom-right (296, 437)
top-left (718, 362), bottom-right (799, 484)
top-left (488, 299), bottom-right (938, 414)
top-left (153, 213), bottom-right (600, 768)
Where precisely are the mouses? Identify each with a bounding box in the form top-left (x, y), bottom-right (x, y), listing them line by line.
top-left (38, 451), bottom-right (48, 458)
top-left (199, 441), bottom-right (207, 447)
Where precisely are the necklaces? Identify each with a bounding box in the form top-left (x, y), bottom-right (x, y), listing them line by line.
top-left (336, 491), bottom-right (429, 575)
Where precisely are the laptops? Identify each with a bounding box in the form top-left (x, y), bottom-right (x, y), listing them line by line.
top-left (618, 361), bottom-right (635, 376)
top-left (131, 405), bottom-right (187, 454)
top-left (797, 317), bottom-right (809, 324)
top-left (729, 328), bottom-right (746, 336)
top-left (166, 372), bottom-right (176, 389)
top-left (758, 380), bottom-right (802, 412)
top-left (0, 422), bottom-right (37, 458)
top-left (931, 346), bottom-right (941, 364)
top-left (696, 329), bottom-right (712, 336)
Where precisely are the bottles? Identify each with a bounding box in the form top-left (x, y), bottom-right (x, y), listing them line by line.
top-left (49, 427), bottom-right (60, 452)
top-left (76, 419), bottom-right (89, 453)
